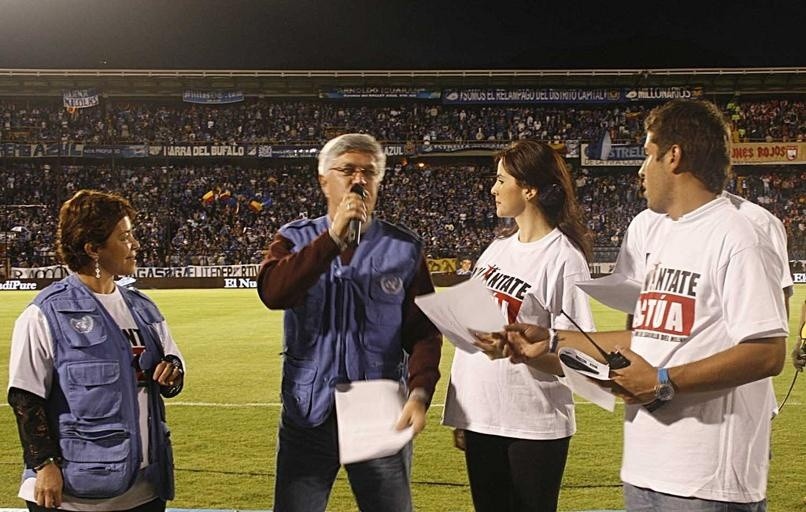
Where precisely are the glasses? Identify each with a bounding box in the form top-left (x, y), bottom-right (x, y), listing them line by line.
top-left (330, 166), bottom-right (381, 175)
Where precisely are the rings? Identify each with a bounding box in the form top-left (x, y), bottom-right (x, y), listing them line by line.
top-left (348, 204), bottom-right (350, 210)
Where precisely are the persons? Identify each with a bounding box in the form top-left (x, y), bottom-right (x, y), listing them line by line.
top-left (490, 97), bottom-right (788, 512)
top-left (790, 298), bottom-right (806, 374)
top-left (439, 141), bottom-right (599, 512)
top-left (2, 96), bottom-right (806, 282)
top-left (257, 133), bottom-right (440, 512)
top-left (721, 189), bottom-right (793, 460)
top-left (5, 190), bottom-right (185, 512)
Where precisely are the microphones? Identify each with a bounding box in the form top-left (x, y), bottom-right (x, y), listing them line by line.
top-left (346, 184), bottom-right (364, 247)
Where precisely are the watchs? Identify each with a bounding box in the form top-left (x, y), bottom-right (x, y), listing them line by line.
top-left (654, 367), bottom-right (675, 403)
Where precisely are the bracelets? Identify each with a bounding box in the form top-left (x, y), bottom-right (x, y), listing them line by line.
top-left (548, 327), bottom-right (558, 353)
top-left (34, 457), bottom-right (54, 470)
